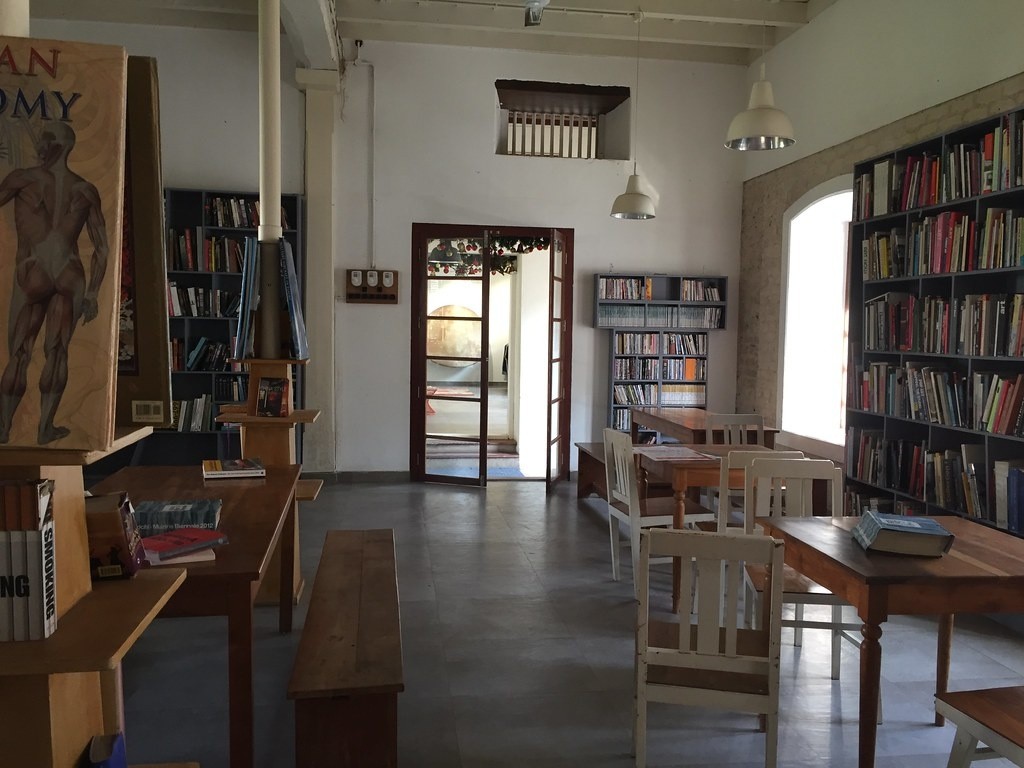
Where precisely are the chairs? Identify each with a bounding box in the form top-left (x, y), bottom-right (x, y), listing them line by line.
top-left (693, 413), bottom-right (883, 724)
top-left (603, 428), bottom-right (715, 581)
top-left (633, 528), bottom-right (786, 768)
top-left (935, 687), bottom-right (1024, 768)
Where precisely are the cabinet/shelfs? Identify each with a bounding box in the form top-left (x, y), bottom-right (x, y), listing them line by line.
top-left (0, 427), bottom-right (187, 768)
top-left (593, 274), bottom-right (728, 445)
top-left (85, 187), bottom-right (307, 476)
top-left (842, 105), bottom-right (1024, 541)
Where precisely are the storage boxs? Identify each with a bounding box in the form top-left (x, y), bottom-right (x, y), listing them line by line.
top-left (85, 493), bottom-right (147, 581)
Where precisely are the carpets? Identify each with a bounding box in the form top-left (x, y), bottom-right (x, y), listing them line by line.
top-left (433, 386), bottom-right (475, 396)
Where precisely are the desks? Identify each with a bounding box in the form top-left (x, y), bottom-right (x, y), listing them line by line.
top-left (632, 445), bottom-right (786, 613)
top-left (756, 515), bottom-right (1024, 768)
top-left (631, 408), bottom-right (780, 529)
top-left (87, 465), bottom-right (303, 768)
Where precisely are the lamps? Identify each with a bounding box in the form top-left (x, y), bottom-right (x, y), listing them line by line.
top-left (429, 238), bottom-right (463, 264)
top-left (610, 13), bottom-right (656, 220)
top-left (724, 0), bottom-right (797, 151)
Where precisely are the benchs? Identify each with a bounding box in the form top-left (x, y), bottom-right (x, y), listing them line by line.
top-left (285, 528), bottom-right (406, 768)
top-left (574, 443), bottom-right (701, 530)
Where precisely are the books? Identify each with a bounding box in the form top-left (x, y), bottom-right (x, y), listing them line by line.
top-left (851, 510), bottom-right (955, 558)
top-left (89, 731), bottom-right (126, 768)
top-left (84, 490), bottom-right (229, 583)
top-left (682, 277), bottom-right (725, 301)
top-left (164, 189), bottom-right (308, 433)
top-left (598, 304), bottom-right (644, 327)
top-left (843, 103), bottom-right (1023, 539)
top-left (680, 306), bottom-right (725, 329)
top-left (614, 330), bottom-right (707, 445)
top-left (647, 305), bottom-right (678, 327)
top-left (1, 477), bottom-right (58, 643)
top-left (598, 277), bottom-right (644, 300)
top-left (202, 458), bottom-right (266, 479)
top-left (646, 278), bottom-right (652, 300)
